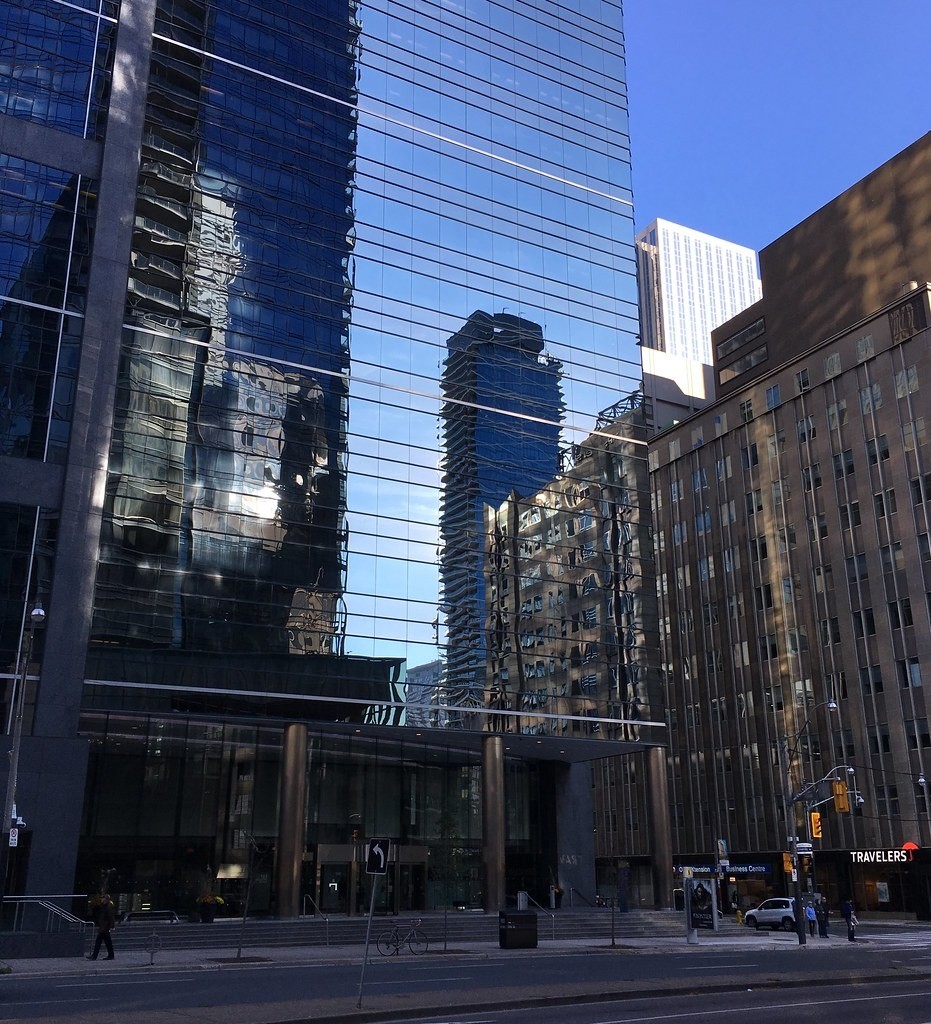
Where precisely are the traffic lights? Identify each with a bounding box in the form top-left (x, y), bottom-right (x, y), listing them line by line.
top-left (812, 812), bottom-right (823, 838)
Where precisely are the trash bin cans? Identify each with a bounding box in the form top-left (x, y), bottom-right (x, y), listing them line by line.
top-left (498, 908), bottom-right (539, 948)
top-left (674, 889), bottom-right (685, 910)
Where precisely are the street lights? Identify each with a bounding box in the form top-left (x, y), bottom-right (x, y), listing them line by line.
top-left (785, 697), bottom-right (855, 944)
top-left (0, 584), bottom-right (45, 931)
top-left (806, 788), bottom-right (865, 935)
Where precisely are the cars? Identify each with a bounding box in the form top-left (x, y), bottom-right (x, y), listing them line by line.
top-left (744, 897), bottom-right (795, 931)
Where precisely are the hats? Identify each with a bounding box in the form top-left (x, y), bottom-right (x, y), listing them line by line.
top-left (807, 900), bottom-right (813, 904)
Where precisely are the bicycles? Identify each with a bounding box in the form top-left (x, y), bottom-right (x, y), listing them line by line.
top-left (377, 918), bottom-right (429, 957)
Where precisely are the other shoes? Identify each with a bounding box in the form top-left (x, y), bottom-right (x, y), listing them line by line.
top-left (104, 955), bottom-right (116, 961)
top-left (84, 954), bottom-right (97, 960)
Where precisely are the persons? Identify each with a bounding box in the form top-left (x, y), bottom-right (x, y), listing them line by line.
top-left (821, 896), bottom-right (832, 934)
top-left (815, 899), bottom-right (828, 938)
top-left (86, 895), bottom-right (116, 961)
top-left (731, 890), bottom-right (738, 914)
top-left (806, 901), bottom-right (817, 937)
top-left (691, 883), bottom-right (713, 911)
top-left (843, 897), bottom-right (857, 942)
